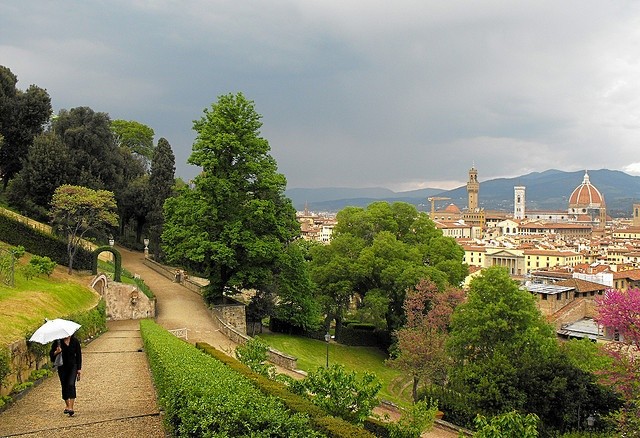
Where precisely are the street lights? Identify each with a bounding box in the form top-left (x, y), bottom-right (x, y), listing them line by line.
top-left (325, 332), bottom-right (331, 366)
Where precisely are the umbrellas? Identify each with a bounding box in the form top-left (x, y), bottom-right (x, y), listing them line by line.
top-left (29, 319), bottom-right (82, 348)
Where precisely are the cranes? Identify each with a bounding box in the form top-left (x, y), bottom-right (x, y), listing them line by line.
top-left (427, 197), bottom-right (451, 219)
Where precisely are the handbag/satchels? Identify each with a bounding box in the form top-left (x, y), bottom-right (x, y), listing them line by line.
top-left (53, 352), bottom-right (63, 366)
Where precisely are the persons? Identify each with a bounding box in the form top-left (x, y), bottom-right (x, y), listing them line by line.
top-left (49, 335), bottom-right (82, 416)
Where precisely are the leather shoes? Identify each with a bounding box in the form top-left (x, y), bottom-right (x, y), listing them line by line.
top-left (64, 409), bottom-right (69, 413)
top-left (69, 410), bottom-right (74, 416)
